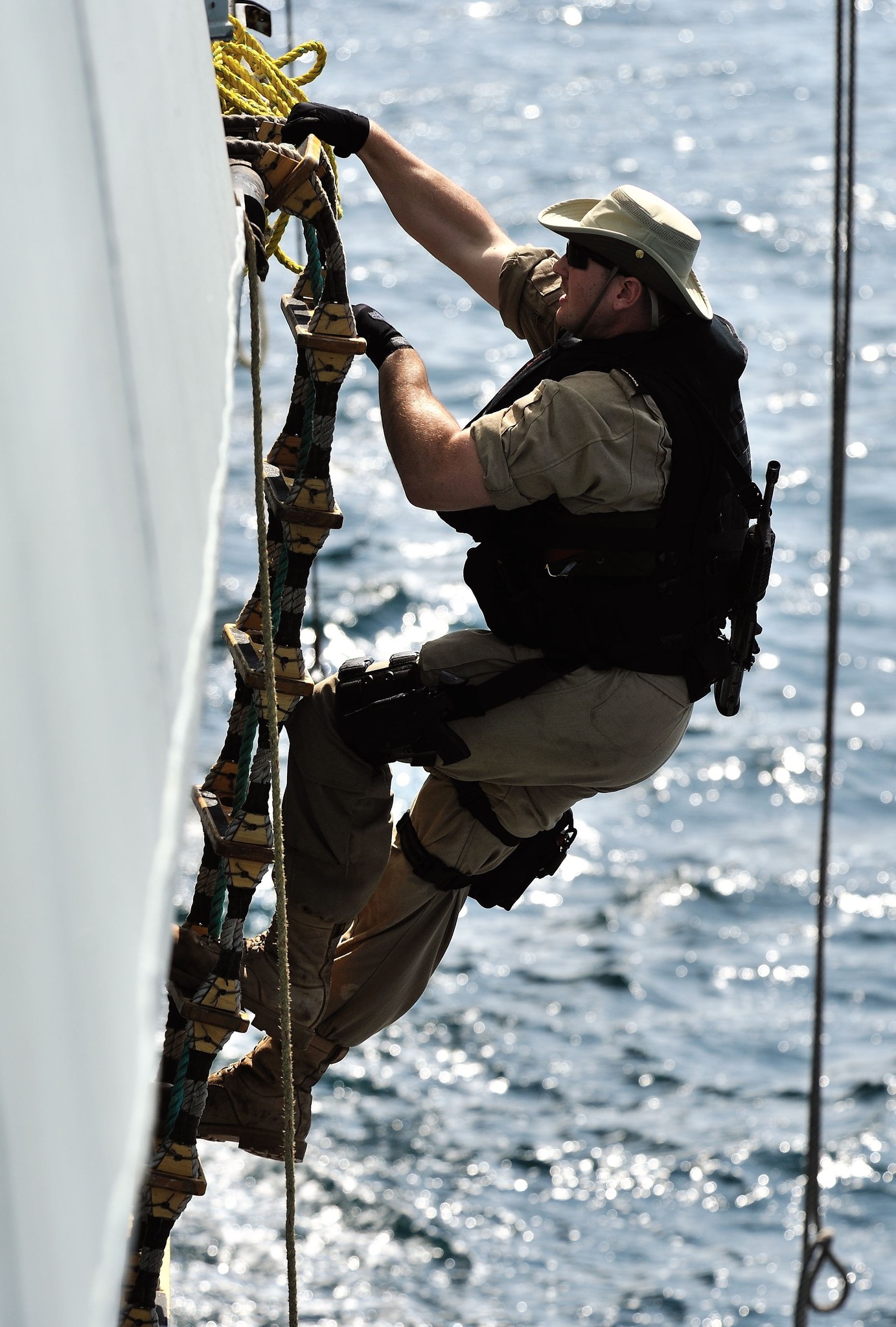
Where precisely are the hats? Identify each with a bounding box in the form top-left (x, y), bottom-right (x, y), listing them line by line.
top-left (538, 188), bottom-right (714, 323)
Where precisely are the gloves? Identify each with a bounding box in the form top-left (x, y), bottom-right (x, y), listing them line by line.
top-left (349, 305), bottom-right (412, 371)
top-left (282, 102), bottom-right (372, 159)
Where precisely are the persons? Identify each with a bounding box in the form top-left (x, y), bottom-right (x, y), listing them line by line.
top-left (197, 101), bottom-right (749, 1161)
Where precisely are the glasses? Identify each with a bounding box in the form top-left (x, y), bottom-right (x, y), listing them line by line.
top-left (565, 243), bottom-right (651, 289)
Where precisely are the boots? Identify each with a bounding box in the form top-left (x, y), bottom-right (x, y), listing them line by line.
top-left (199, 1027), bottom-right (354, 1164)
top-left (238, 907), bottom-right (341, 1049)
